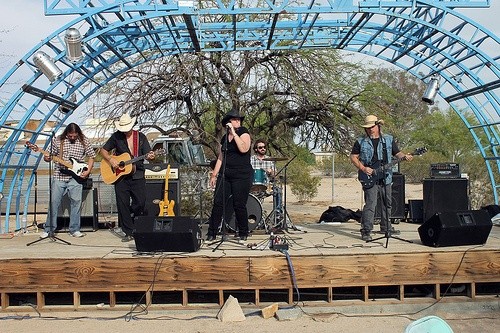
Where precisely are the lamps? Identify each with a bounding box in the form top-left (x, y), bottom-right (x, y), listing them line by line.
top-left (422, 73), bottom-right (441, 105)
top-left (64, 27), bottom-right (84, 64)
top-left (32, 51), bottom-right (63, 85)
top-left (58, 93), bottom-right (76, 114)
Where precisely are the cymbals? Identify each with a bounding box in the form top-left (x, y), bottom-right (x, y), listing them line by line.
top-left (262, 158), bottom-right (288, 161)
top-left (210, 160), bottom-right (218, 169)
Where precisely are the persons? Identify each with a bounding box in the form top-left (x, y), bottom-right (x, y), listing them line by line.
top-left (40, 122), bottom-right (97, 238)
top-left (350, 115), bottom-right (413, 240)
top-left (251, 139), bottom-right (277, 202)
top-left (206, 110), bottom-right (252, 240)
top-left (100, 114), bottom-right (156, 242)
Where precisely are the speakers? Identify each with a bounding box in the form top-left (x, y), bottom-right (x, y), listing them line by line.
top-left (143, 179), bottom-right (180, 217)
top-left (421, 178), bottom-right (467, 221)
top-left (418, 209), bottom-right (493, 247)
top-left (57, 188), bottom-right (98, 232)
top-left (133, 216), bottom-right (202, 252)
top-left (372, 174), bottom-right (405, 219)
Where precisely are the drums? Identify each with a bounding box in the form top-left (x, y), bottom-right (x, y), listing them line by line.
top-left (249, 167), bottom-right (268, 192)
top-left (225, 193), bottom-right (263, 233)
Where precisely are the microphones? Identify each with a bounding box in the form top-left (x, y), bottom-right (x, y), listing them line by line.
top-left (226, 121), bottom-right (231, 128)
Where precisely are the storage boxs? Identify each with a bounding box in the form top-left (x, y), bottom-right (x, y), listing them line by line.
top-left (422, 179), bottom-right (471, 221)
top-left (408, 200), bottom-right (423, 222)
top-left (146, 180), bottom-right (180, 216)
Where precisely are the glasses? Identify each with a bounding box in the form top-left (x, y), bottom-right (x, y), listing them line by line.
top-left (256, 146), bottom-right (266, 149)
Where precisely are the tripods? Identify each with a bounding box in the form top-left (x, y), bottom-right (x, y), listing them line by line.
top-left (366, 126), bottom-right (413, 248)
top-left (26, 134), bottom-right (71, 245)
top-left (209, 128), bottom-right (307, 252)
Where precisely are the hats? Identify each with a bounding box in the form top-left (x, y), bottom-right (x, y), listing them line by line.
top-left (222, 111), bottom-right (244, 127)
top-left (115, 114), bottom-right (136, 132)
top-left (360, 115), bottom-right (384, 127)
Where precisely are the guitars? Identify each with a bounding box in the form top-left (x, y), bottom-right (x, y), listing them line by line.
top-left (358, 146), bottom-right (428, 189)
top-left (25, 141), bottom-right (88, 185)
top-left (100, 148), bottom-right (167, 185)
top-left (157, 165), bottom-right (175, 216)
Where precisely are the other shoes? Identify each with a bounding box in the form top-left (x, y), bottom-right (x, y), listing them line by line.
top-left (362, 235), bottom-right (372, 241)
top-left (206, 235), bottom-right (216, 241)
top-left (241, 236), bottom-right (247, 240)
top-left (121, 236), bottom-right (134, 242)
top-left (380, 229), bottom-right (400, 235)
top-left (70, 231), bottom-right (85, 237)
top-left (39, 232), bottom-right (55, 238)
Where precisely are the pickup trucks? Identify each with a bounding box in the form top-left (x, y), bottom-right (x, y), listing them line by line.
top-left (146, 132), bottom-right (221, 196)
